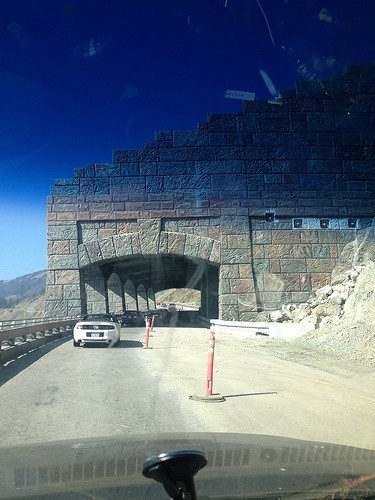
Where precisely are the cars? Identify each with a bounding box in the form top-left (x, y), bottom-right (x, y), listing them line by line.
top-left (121, 310), bottom-right (144, 327)
top-left (143, 302), bottom-right (176, 327)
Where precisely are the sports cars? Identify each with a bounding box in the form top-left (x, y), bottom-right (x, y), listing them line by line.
top-left (74, 314), bottom-right (122, 348)
